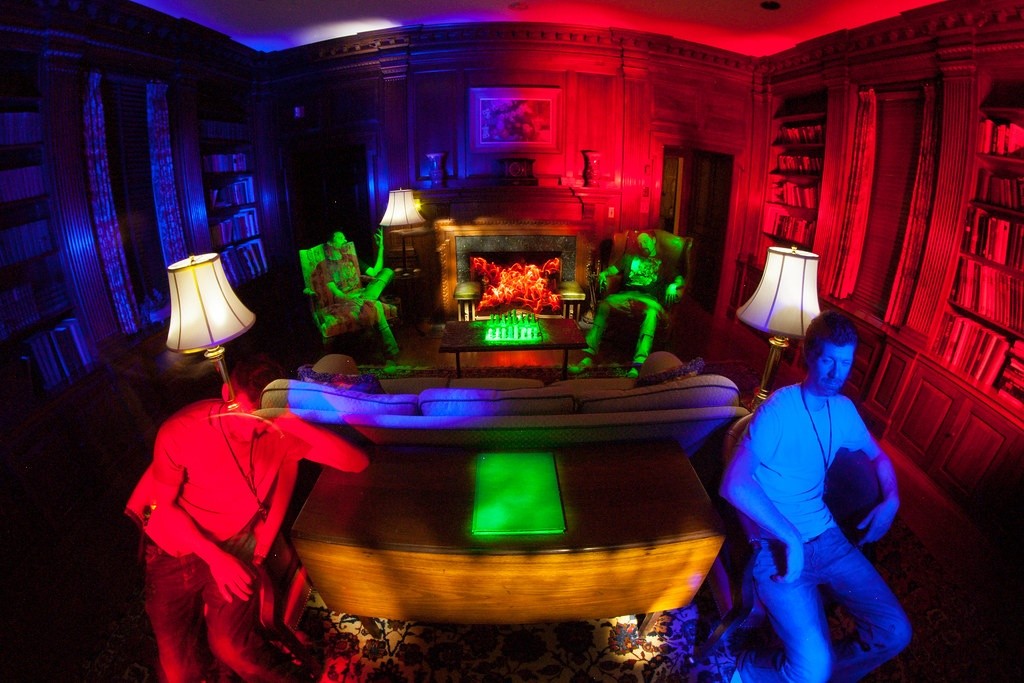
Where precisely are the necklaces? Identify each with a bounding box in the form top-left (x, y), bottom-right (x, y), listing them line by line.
top-left (219, 403), bottom-right (272, 522)
top-left (800, 383), bottom-right (832, 471)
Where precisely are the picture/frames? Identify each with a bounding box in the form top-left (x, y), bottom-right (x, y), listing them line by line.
top-left (464, 85), bottom-right (564, 156)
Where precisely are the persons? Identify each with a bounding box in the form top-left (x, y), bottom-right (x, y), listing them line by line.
top-left (314, 231), bottom-right (402, 365)
top-left (126, 350), bottom-right (370, 683)
top-left (717, 310), bottom-right (913, 683)
top-left (568, 229), bottom-right (685, 379)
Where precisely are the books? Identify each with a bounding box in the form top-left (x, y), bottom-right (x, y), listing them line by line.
top-left (931, 119), bottom-right (1024, 419)
top-left (0, 109), bottom-right (98, 424)
top-left (199, 146), bottom-right (269, 288)
top-left (760, 122), bottom-right (828, 250)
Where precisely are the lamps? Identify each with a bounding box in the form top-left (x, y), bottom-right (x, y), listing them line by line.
top-left (164, 252), bottom-right (258, 411)
top-left (380, 189), bottom-right (423, 277)
top-left (738, 246), bottom-right (829, 416)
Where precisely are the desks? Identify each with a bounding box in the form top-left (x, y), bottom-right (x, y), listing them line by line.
top-left (439, 320), bottom-right (589, 380)
top-left (391, 266), bottom-right (424, 338)
top-left (295, 448), bottom-right (724, 639)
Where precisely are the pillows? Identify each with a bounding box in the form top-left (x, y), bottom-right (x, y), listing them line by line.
top-left (298, 365), bottom-right (384, 395)
top-left (633, 357), bottom-right (704, 389)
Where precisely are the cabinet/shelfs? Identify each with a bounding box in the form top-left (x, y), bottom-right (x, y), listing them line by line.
top-left (0, 63), bottom-right (269, 412)
top-left (731, 77), bottom-right (1024, 537)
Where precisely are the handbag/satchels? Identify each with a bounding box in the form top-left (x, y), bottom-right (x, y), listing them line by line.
top-left (822, 447), bottom-right (883, 544)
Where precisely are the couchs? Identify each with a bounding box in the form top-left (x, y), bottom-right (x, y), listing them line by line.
top-left (596, 229), bottom-right (697, 354)
top-left (259, 352), bottom-right (749, 454)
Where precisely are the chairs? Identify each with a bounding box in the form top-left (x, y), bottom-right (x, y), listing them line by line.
top-left (687, 411), bottom-right (876, 676)
top-left (124, 450), bottom-right (315, 672)
top-left (298, 241), bottom-right (397, 356)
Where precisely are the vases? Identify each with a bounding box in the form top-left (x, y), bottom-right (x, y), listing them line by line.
top-left (581, 150), bottom-right (603, 187)
top-left (426, 152), bottom-right (447, 191)
